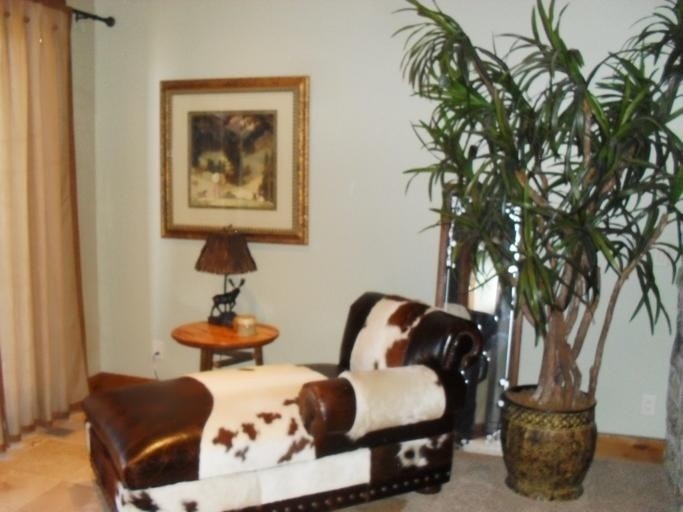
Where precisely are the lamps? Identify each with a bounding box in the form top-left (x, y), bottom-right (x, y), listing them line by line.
top-left (195, 224), bottom-right (257, 323)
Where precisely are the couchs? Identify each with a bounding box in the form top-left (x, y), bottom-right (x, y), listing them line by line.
top-left (81, 293), bottom-right (482, 512)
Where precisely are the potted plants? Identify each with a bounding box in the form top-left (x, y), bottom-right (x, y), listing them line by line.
top-left (389, 1), bottom-right (683, 501)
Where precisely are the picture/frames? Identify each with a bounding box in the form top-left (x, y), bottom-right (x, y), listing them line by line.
top-left (159, 75), bottom-right (310, 244)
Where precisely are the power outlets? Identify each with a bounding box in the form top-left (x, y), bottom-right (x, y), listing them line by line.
top-left (151, 341), bottom-right (163, 359)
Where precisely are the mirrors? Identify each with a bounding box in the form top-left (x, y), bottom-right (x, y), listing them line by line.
top-left (436, 181), bottom-right (534, 436)
